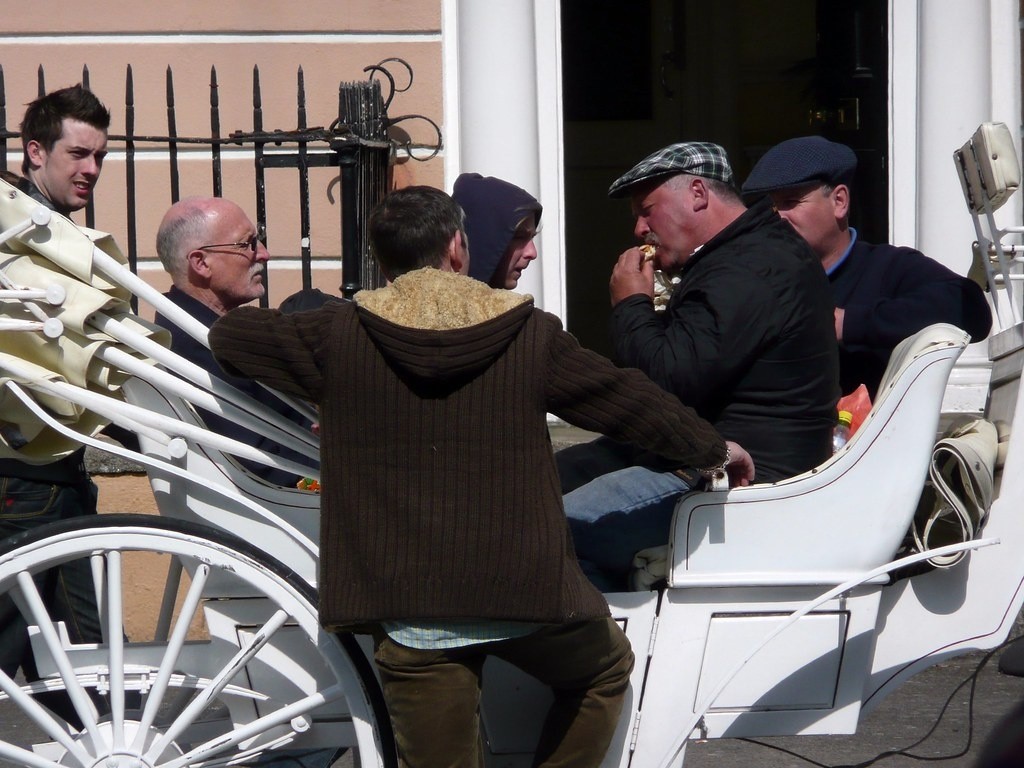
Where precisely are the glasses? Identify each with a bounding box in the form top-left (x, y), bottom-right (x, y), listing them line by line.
top-left (188, 222), bottom-right (267, 264)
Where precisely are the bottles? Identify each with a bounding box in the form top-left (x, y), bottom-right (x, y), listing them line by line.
top-left (832, 410), bottom-right (853, 456)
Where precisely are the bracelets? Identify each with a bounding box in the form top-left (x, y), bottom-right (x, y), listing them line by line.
top-left (695, 448), bottom-right (732, 474)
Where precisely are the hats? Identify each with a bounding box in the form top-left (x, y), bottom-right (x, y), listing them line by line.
top-left (607, 142), bottom-right (738, 198)
top-left (740, 136), bottom-right (858, 194)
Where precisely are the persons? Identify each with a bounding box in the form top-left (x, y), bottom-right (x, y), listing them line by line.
top-left (0, 85), bottom-right (144, 741)
top-left (209, 185), bottom-right (755, 768)
top-left (739, 134), bottom-right (992, 407)
top-left (551, 141), bottom-right (841, 592)
top-left (449, 173), bottom-right (541, 294)
top-left (156, 195), bottom-right (322, 488)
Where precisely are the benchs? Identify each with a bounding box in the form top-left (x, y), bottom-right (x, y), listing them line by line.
top-left (631, 321), bottom-right (975, 587)
top-left (119, 374), bottom-right (394, 600)
